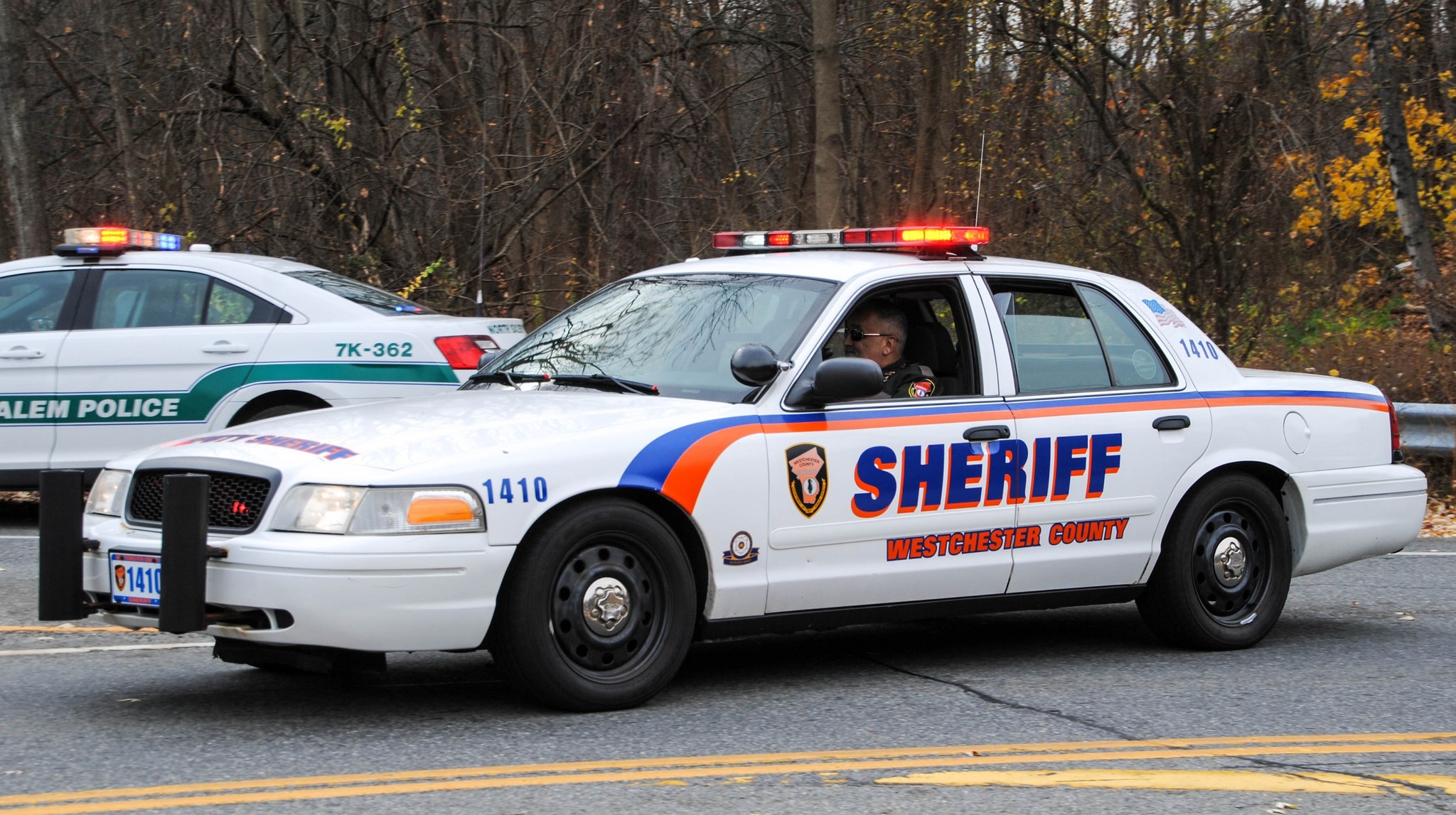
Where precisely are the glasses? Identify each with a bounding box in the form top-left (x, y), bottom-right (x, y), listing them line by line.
top-left (844, 328), bottom-right (899, 343)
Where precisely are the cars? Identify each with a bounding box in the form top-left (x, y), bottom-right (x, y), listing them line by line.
top-left (0, 219), bottom-right (532, 494)
top-left (85, 226), bottom-right (1435, 709)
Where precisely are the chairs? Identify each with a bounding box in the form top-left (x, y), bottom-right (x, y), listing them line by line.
top-left (112, 288), bottom-right (148, 329)
top-left (901, 320), bottom-right (968, 397)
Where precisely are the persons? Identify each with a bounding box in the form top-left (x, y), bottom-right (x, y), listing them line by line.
top-left (822, 295), bottom-right (944, 402)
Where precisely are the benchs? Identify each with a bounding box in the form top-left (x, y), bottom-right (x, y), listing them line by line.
top-left (1014, 354), bottom-right (1165, 395)
